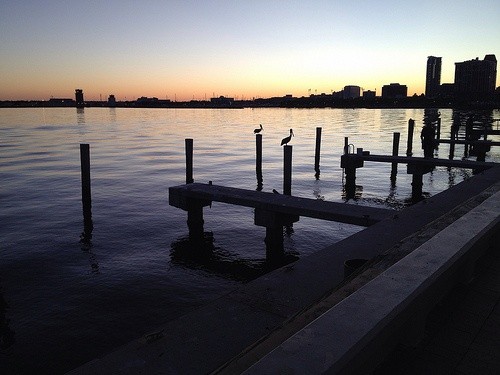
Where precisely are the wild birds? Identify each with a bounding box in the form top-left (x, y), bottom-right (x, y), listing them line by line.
top-left (254, 124), bottom-right (263, 134)
top-left (281, 129), bottom-right (294, 146)
